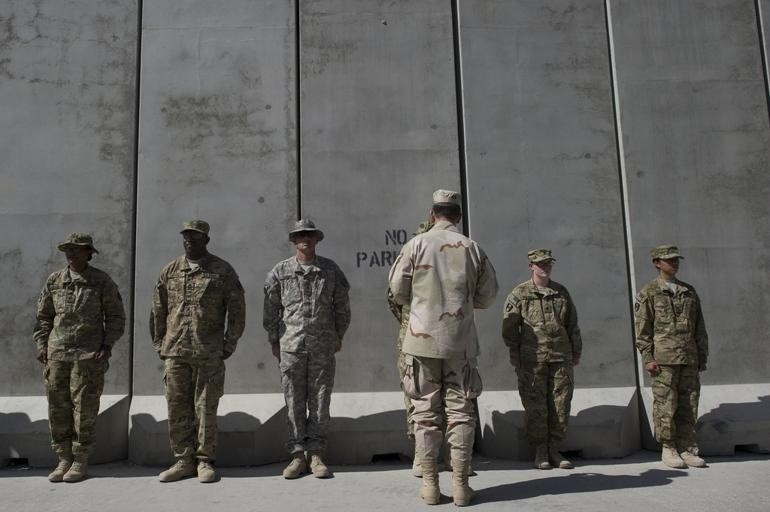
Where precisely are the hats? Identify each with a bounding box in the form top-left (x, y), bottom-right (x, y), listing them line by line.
top-left (432, 190), bottom-right (461, 206)
top-left (57, 232), bottom-right (99, 254)
top-left (412, 220), bottom-right (435, 235)
top-left (179, 219), bottom-right (210, 234)
top-left (528, 248), bottom-right (556, 263)
top-left (289, 220), bottom-right (324, 241)
top-left (651, 246), bottom-right (684, 261)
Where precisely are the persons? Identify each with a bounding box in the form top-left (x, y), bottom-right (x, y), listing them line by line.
top-left (386, 217), bottom-right (475, 479)
top-left (501, 247), bottom-right (584, 470)
top-left (145, 217), bottom-right (246, 485)
top-left (633, 244), bottom-right (710, 470)
top-left (31, 230), bottom-right (126, 484)
top-left (259, 217), bottom-right (354, 481)
top-left (384, 189), bottom-right (501, 507)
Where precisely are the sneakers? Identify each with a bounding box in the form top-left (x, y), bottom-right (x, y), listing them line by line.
top-left (303, 450), bottom-right (330, 478)
top-left (420, 485), bottom-right (441, 505)
top-left (412, 461), bottom-right (438, 477)
top-left (679, 450), bottom-right (705, 467)
top-left (48, 458), bottom-right (88, 483)
top-left (449, 460), bottom-right (473, 476)
top-left (534, 444), bottom-right (552, 469)
top-left (158, 457), bottom-right (198, 483)
top-left (661, 442), bottom-right (687, 468)
top-left (197, 460), bottom-right (218, 483)
top-left (282, 451), bottom-right (307, 479)
top-left (453, 485), bottom-right (474, 506)
top-left (548, 447), bottom-right (572, 469)
